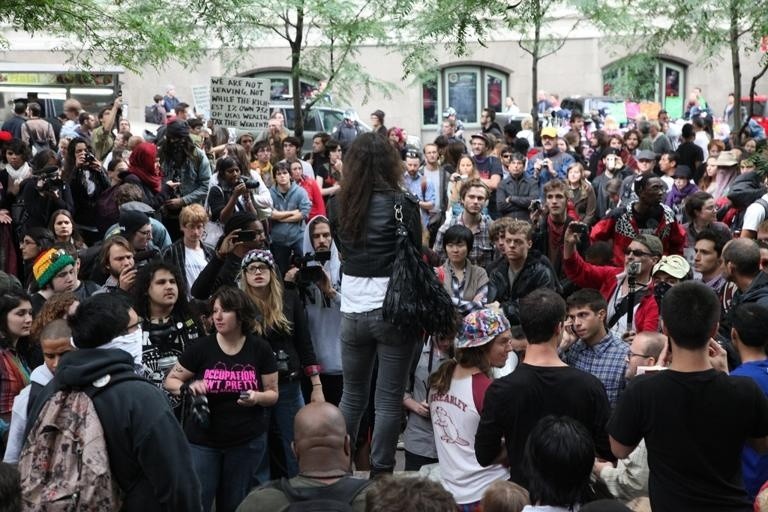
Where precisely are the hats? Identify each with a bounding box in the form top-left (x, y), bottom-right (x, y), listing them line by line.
top-left (239, 248), bottom-right (277, 266)
top-left (632, 232), bottom-right (665, 258)
top-left (235, 129), bottom-right (258, 143)
top-left (116, 199), bottom-right (156, 216)
top-left (470, 130), bottom-right (492, 143)
top-left (121, 211), bottom-right (153, 243)
top-left (539, 126), bottom-right (559, 139)
top-left (453, 307), bottom-right (514, 350)
top-left (599, 147), bottom-right (620, 160)
top-left (711, 149), bottom-right (740, 168)
top-left (650, 252), bottom-right (697, 284)
top-left (667, 164), bottom-right (694, 179)
top-left (371, 109), bottom-right (385, 122)
top-left (636, 149), bottom-right (657, 162)
top-left (30, 246), bottom-right (77, 292)
top-left (166, 118), bottom-right (192, 138)
top-left (439, 106), bottom-right (459, 118)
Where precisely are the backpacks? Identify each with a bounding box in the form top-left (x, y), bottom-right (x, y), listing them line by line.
top-left (13, 386), bottom-right (120, 512)
top-left (280, 484), bottom-right (360, 512)
top-left (29, 138), bottom-right (52, 160)
top-left (9, 198), bottom-right (33, 240)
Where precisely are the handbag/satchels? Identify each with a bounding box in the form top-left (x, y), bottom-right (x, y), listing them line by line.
top-left (168, 381), bottom-right (215, 447)
top-left (427, 213), bottom-right (443, 234)
top-left (380, 234), bottom-right (458, 340)
top-left (198, 219), bottom-right (226, 252)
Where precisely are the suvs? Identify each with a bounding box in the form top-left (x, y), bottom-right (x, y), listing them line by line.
top-left (264, 104), bottom-right (421, 152)
top-left (556, 95), bottom-right (647, 115)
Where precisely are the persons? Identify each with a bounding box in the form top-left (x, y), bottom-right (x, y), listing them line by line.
top-left (2, 86), bottom-right (768, 512)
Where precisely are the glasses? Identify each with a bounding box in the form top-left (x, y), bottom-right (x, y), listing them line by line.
top-left (501, 153), bottom-right (515, 158)
top-left (621, 246), bottom-right (658, 259)
top-left (403, 151), bottom-right (420, 159)
top-left (35, 248), bottom-right (68, 272)
top-left (136, 227), bottom-right (152, 237)
top-left (241, 263), bottom-right (274, 275)
top-left (673, 176), bottom-right (690, 179)
top-left (625, 350), bottom-right (650, 361)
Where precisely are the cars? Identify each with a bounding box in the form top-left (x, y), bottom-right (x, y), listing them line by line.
top-left (731, 94), bottom-right (768, 136)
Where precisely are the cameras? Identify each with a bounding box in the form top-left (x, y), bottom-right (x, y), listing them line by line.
top-left (626, 261), bottom-right (644, 275)
top-left (290, 251), bottom-right (332, 285)
top-left (32, 166), bottom-right (67, 191)
top-left (84, 152), bottom-right (95, 162)
top-left (453, 174), bottom-right (469, 181)
top-left (236, 174), bottom-right (261, 190)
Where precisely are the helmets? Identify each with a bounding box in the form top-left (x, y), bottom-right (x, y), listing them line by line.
top-left (343, 108), bottom-right (359, 121)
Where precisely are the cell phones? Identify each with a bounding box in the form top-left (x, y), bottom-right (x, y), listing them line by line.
top-left (232, 230), bottom-right (256, 243)
top-left (571, 224), bottom-right (589, 234)
top-left (117, 89), bottom-right (123, 98)
